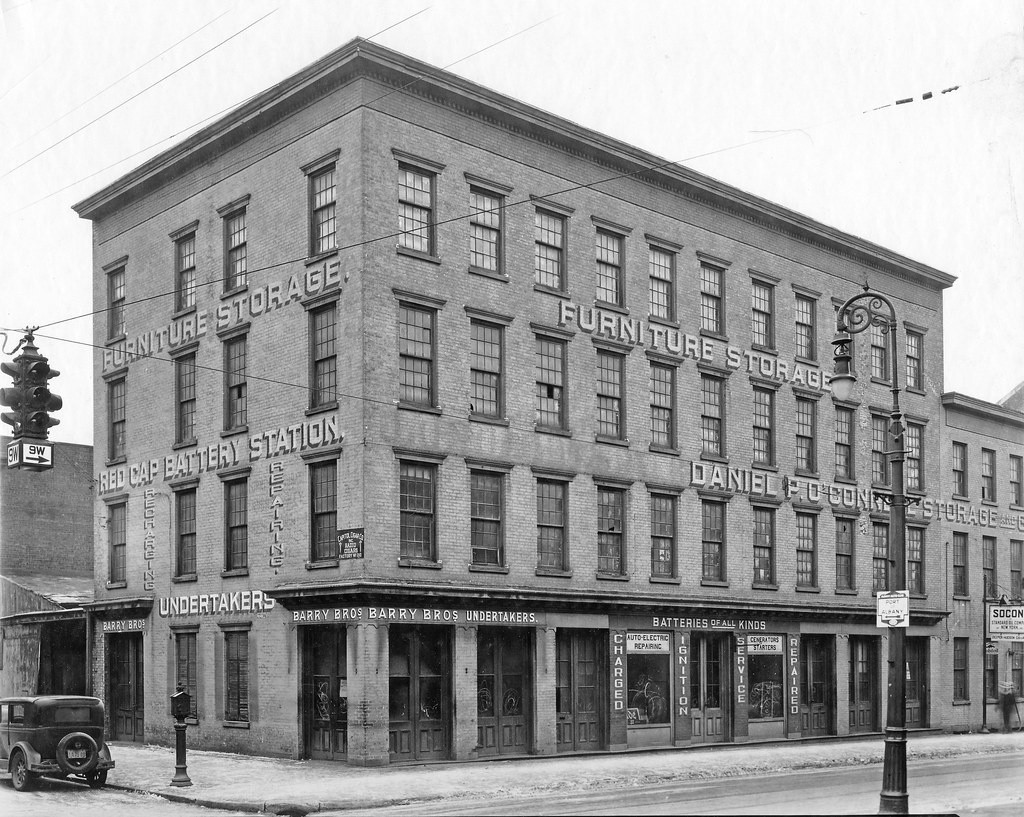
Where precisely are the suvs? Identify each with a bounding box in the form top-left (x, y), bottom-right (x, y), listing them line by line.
top-left (1, 695), bottom-right (116, 791)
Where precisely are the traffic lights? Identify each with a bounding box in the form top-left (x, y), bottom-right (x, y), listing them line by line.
top-left (0, 360), bottom-right (64, 438)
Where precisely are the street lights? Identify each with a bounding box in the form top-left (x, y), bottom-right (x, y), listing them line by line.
top-left (825, 289), bottom-right (913, 816)
